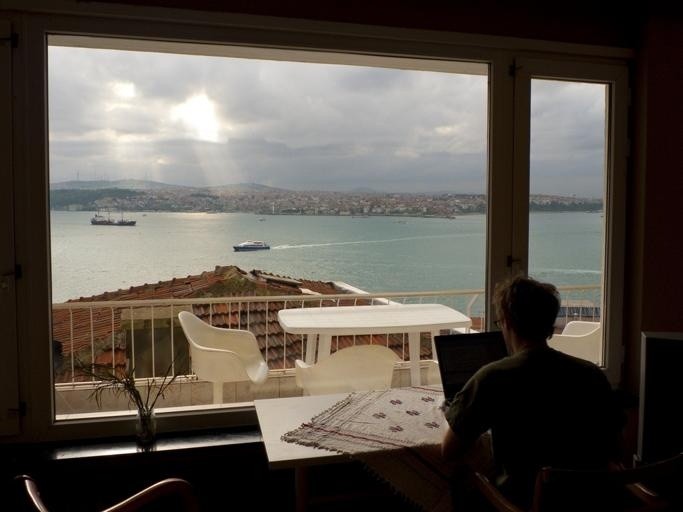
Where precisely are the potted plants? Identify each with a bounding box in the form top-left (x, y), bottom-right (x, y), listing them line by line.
top-left (66, 351), bottom-right (189, 450)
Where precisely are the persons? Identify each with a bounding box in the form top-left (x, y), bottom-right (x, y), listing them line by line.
top-left (441, 276), bottom-right (621, 509)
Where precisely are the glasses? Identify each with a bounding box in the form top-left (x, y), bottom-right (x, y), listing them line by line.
top-left (493, 317), bottom-right (503, 328)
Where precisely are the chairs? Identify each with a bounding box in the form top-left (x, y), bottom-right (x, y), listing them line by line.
top-left (11, 469), bottom-right (203, 510)
top-left (175, 309), bottom-right (271, 406)
top-left (546, 319), bottom-right (605, 368)
top-left (471, 439), bottom-right (679, 511)
top-left (293, 343), bottom-right (401, 399)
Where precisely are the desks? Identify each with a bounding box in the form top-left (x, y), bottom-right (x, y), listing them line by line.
top-left (276, 301), bottom-right (473, 387)
top-left (251, 383), bottom-right (498, 510)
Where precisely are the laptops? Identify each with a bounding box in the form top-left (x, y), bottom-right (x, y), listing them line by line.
top-left (434, 330), bottom-right (510, 407)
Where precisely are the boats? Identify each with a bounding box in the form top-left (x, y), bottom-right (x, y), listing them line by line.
top-left (232, 239), bottom-right (271, 252)
top-left (86, 206), bottom-right (137, 227)
top-left (258, 217), bottom-right (266, 221)
